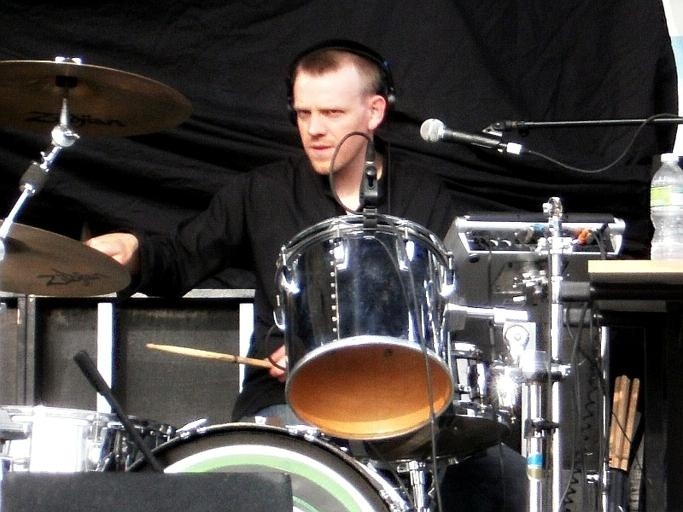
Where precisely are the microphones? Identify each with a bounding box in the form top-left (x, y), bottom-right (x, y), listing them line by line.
top-left (420, 118), bottom-right (525, 157)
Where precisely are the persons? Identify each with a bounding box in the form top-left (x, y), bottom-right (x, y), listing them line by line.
top-left (80, 39), bottom-right (531, 512)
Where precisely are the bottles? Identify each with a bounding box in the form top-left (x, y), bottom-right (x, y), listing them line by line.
top-left (648, 153), bottom-right (683, 261)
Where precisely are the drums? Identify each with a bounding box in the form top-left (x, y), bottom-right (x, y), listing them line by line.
top-left (129, 421), bottom-right (416, 512)
top-left (350, 343), bottom-right (518, 465)
top-left (0, 405), bottom-right (175, 472)
top-left (274, 212), bottom-right (456, 440)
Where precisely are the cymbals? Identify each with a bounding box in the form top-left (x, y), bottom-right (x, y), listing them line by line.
top-left (1, 60), bottom-right (195, 140)
top-left (0, 220), bottom-right (131, 297)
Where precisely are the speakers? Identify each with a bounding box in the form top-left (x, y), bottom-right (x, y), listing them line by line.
top-left (0, 472), bottom-right (292, 512)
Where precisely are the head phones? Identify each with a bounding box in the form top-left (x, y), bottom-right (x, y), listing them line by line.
top-left (286, 39), bottom-right (396, 126)
